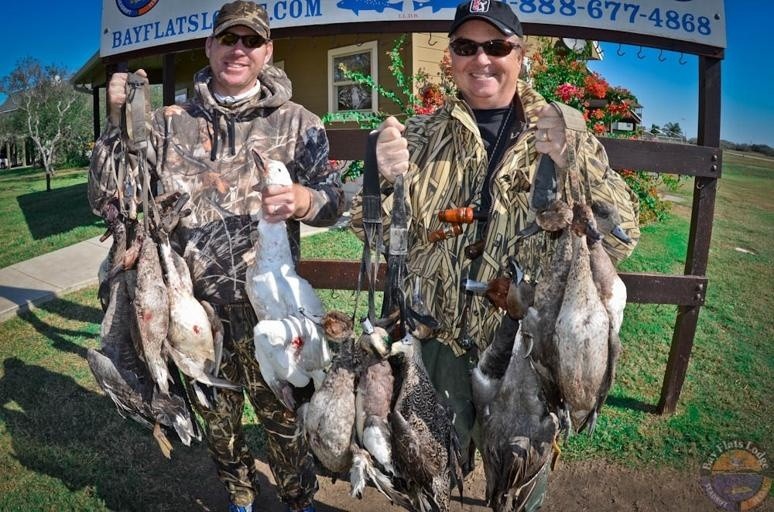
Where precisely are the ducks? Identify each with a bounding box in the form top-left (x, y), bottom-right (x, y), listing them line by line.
top-left (242, 147), bottom-right (334, 413)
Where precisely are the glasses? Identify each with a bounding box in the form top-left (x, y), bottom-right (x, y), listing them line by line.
top-left (450, 39), bottom-right (521, 56)
top-left (215, 32), bottom-right (265, 48)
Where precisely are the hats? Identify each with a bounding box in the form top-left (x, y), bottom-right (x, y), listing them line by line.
top-left (213, 1), bottom-right (271, 40)
top-left (448, 0), bottom-right (523, 38)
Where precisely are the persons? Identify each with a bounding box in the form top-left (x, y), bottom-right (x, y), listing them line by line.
top-left (370, 0), bottom-right (641, 512)
top-left (87, 0), bottom-right (346, 512)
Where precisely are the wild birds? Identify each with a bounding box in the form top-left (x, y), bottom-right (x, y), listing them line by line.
top-left (298, 199), bottom-right (632, 512)
top-left (86, 125), bottom-right (245, 458)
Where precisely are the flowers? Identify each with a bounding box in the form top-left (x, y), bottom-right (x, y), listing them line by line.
top-left (322, 34), bottom-right (693, 232)
